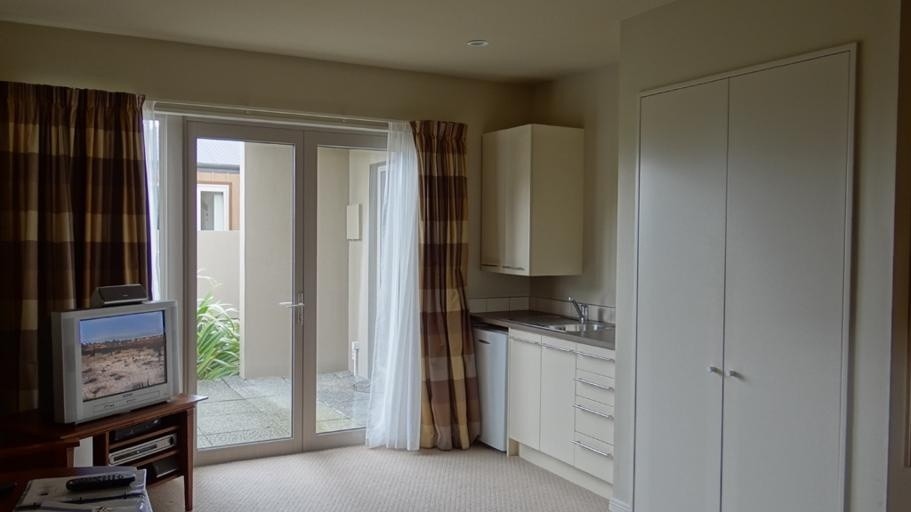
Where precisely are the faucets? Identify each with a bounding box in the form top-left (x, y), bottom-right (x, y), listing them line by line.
top-left (568, 296), bottom-right (588, 323)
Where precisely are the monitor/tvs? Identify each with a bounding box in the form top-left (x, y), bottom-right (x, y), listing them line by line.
top-left (50, 300), bottom-right (180, 427)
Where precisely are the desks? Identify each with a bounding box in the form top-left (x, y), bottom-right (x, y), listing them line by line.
top-left (0, 466), bottom-right (154, 512)
top-left (59, 393), bottom-right (208, 511)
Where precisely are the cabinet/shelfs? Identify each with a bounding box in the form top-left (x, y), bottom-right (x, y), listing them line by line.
top-left (506, 327), bottom-right (616, 499)
top-left (480, 123), bottom-right (585, 277)
top-left (630, 47), bottom-right (851, 511)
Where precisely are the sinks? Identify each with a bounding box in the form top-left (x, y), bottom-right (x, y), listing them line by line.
top-left (522, 319), bottom-right (606, 334)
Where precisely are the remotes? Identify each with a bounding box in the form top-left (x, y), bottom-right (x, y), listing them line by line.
top-left (66, 473), bottom-right (136, 491)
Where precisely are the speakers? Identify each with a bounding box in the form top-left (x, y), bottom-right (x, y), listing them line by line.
top-left (91, 284), bottom-right (148, 307)
